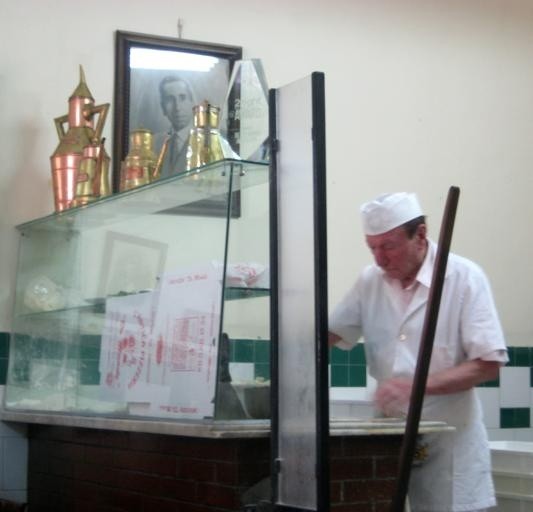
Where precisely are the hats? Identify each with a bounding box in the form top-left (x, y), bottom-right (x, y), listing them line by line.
top-left (357, 192), bottom-right (423, 236)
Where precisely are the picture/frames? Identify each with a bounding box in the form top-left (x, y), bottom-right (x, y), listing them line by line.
top-left (111, 30), bottom-right (244, 219)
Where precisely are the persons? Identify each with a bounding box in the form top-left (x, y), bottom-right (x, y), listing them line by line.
top-left (142, 73), bottom-right (240, 178)
top-left (324, 190), bottom-right (513, 511)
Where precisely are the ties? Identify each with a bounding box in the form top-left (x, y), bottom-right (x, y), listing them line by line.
top-left (168, 134), bottom-right (179, 175)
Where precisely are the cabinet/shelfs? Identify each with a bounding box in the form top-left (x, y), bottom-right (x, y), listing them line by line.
top-left (3, 158), bottom-right (271, 425)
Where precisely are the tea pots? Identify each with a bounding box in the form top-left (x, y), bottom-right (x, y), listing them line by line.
top-left (69, 137), bottom-right (111, 214)
top-left (120, 130), bottom-right (171, 193)
top-left (183, 99), bottom-right (226, 186)
